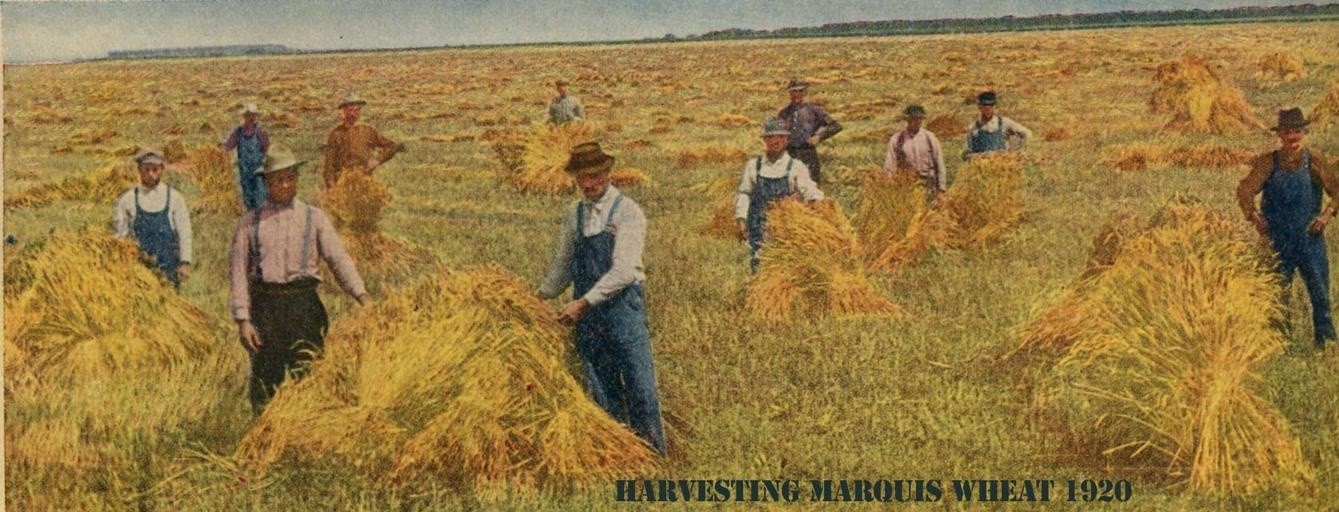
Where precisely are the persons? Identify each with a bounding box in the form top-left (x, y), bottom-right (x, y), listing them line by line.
top-left (782, 78), bottom-right (845, 183)
top-left (961, 92), bottom-right (1035, 152)
top-left (233, 144), bottom-right (371, 422)
top-left (115, 146), bottom-right (193, 296)
top-left (1236, 113), bottom-right (1338, 351)
top-left (537, 147), bottom-right (669, 458)
top-left (324, 97), bottom-right (405, 197)
top-left (882, 106), bottom-right (945, 197)
top-left (228, 100), bottom-right (270, 213)
top-left (550, 75), bottom-right (586, 128)
top-left (735, 119), bottom-right (835, 279)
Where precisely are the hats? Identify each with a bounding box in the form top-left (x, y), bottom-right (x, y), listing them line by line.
top-left (1271, 106), bottom-right (1310, 131)
top-left (241, 103), bottom-right (262, 115)
top-left (759, 117), bottom-right (791, 137)
top-left (787, 80), bottom-right (809, 91)
top-left (134, 149), bottom-right (164, 165)
top-left (975, 92), bottom-right (997, 106)
top-left (564, 142), bottom-right (616, 176)
top-left (338, 94), bottom-right (367, 108)
top-left (903, 104), bottom-right (927, 119)
top-left (556, 78), bottom-right (570, 85)
top-left (253, 143), bottom-right (308, 175)
